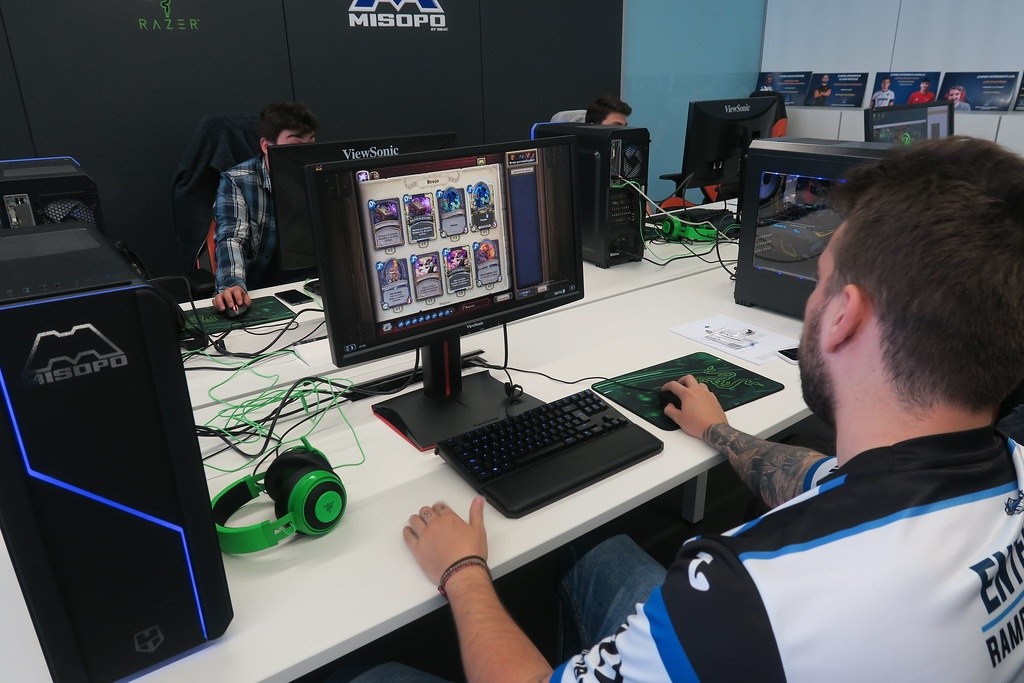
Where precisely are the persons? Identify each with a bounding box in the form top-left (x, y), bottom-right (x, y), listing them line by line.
top-left (908, 78), bottom-right (936, 104)
top-left (871, 77), bottom-right (895, 109)
top-left (813, 74), bottom-right (832, 107)
top-left (760, 74), bottom-right (772, 91)
top-left (945, 86), bottom-right (972, 111)
top-left (212, 101), bottom-right (320, 314)
top-left (320, 135), bottom-right (1024, 683)
top-left (584, 94), bottom-right (654, 216)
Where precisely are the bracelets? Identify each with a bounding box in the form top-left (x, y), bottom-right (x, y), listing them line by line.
top-left (437, 555), bottom-right (493, 597)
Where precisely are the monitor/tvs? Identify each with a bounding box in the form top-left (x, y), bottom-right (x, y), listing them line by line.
top-left (863, 100), bottom-right (954, 145)
top-left (680, 95), bottom-right (778, 189)
top-left (268, 132), bottom-right (585, 451)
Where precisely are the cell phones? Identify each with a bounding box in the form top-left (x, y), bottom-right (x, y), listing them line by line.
top-left (777, 347), bottom-right (799, 365)
top-left (275, 289), bottom-right (314, 306)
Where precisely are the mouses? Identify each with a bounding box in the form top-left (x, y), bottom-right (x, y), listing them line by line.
top-left (657, 390), bottom-right (682, 411)
top-left (225, 302), bottom-right (248, 318)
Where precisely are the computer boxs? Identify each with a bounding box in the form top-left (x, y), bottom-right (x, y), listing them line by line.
top-left (0, 156), bottom-right (234, 683)
top-left (532, 120), bottom-right (652, 268)
top-left (734, 137), bottom-right (899, 322)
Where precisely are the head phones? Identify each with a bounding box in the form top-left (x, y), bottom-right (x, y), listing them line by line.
top-left (661, 216), bottom-right (717, 242)
top-left (212, 436), bottom-right (348, 555)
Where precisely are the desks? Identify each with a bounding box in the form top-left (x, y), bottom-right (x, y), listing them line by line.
top-left (0, 198), bottom-right (813, 683)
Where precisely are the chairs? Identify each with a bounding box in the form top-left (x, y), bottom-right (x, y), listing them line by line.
top-left (164, 113), bottom-right (262, 300)
top-left (550, 110), bottom-right (588, 126)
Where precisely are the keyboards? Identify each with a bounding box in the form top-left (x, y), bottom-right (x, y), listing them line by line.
top-left (303, 280), bottom-right (321, 296)
top-left (646, 208), bottom-right (728, 225)
top-left (435, 389), bottom-right (629, 488)
